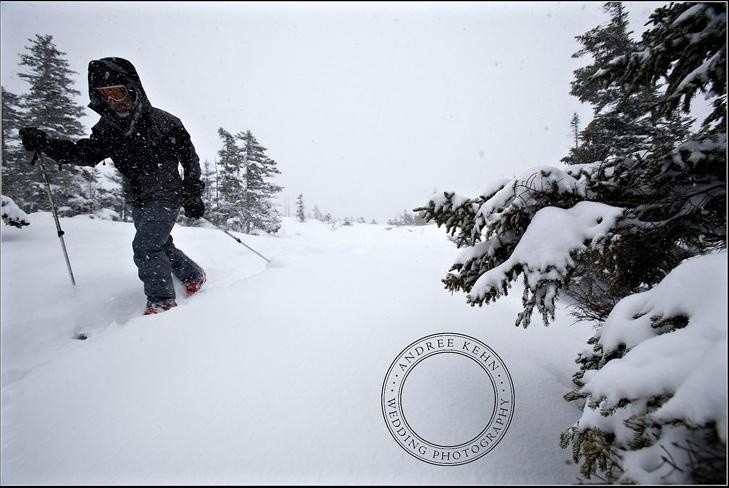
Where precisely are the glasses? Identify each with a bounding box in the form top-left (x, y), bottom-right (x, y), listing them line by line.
top-left (94, 83), bottom-right (130, 103)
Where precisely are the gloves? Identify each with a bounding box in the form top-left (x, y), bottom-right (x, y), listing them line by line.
top-left (181, 195), bottom-right (205, 220)
top-left (18, 126), bottom-right (49, 152)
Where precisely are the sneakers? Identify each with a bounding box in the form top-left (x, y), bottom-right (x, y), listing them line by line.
top-left (183, 273), bottom-right (207, 297)
top-left (143, 298), bottom-right (177, 317)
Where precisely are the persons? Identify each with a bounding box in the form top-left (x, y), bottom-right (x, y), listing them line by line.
top-left (19, 57), bottom-right (206, 314)
